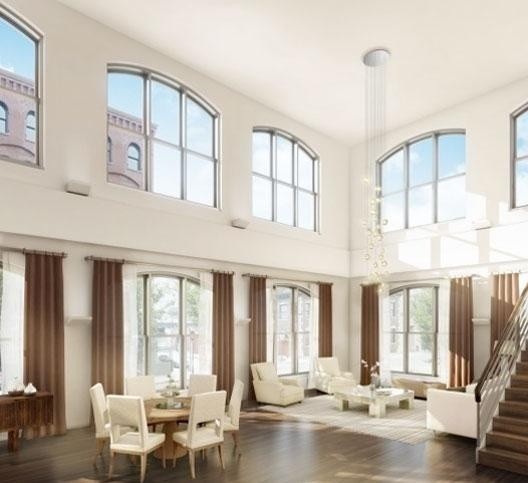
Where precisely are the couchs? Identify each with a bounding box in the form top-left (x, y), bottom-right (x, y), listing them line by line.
top-left (251, 362), bottom-right (304, 405)
top-left (311, 356), bottom-right (356, 394)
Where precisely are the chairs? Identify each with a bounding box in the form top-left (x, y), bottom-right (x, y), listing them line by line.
top-left (172, 390), bottom-right (228, 478)
top-left (185, 373), bottom-right (218, 392)
top-left (123, 377), bottom-right (159, 398)
top-left (200, 376), bottom-right (244, 458)
top-left (106, 392), bottom-right (166, 483)
top-left (90, 381), bottom-right (138, 466)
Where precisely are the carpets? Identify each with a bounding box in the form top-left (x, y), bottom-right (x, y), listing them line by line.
top-left (259, 395), bottom-right (441, 445)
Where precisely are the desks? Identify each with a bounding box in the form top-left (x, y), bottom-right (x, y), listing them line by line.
top-left (335, 384), bottom-right (415, 417)
top-left (139, 395), bottom-right (201, 459)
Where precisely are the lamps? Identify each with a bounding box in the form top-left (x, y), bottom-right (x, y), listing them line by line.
top-left (360, 47), bottom-right (396, 294)
